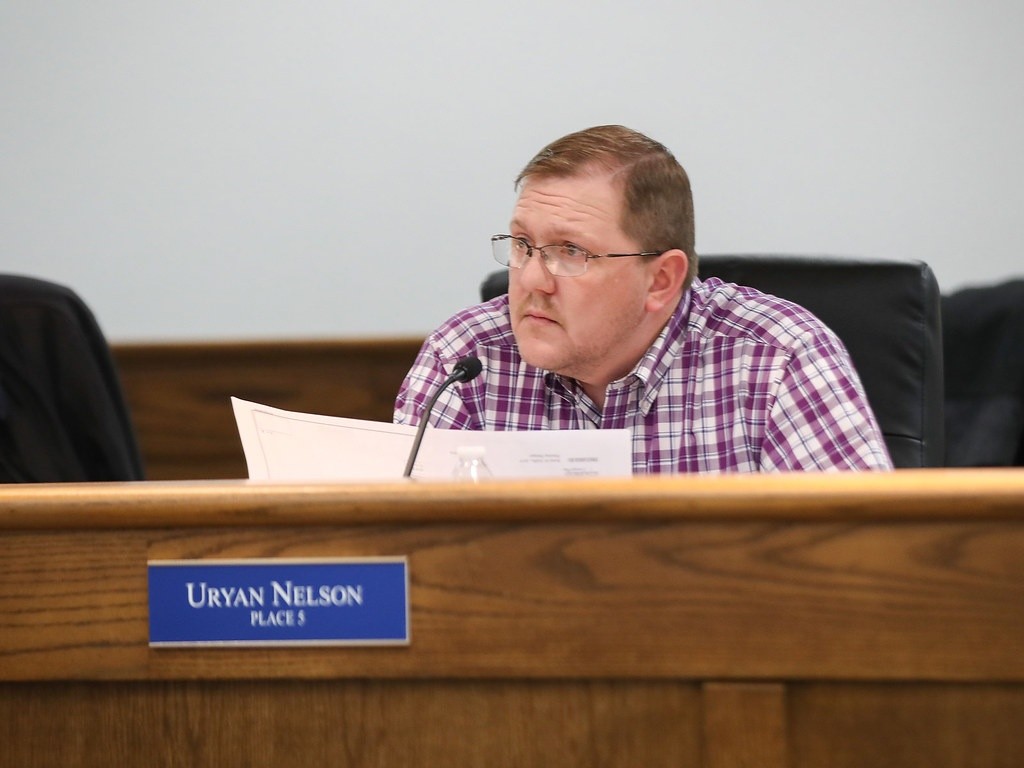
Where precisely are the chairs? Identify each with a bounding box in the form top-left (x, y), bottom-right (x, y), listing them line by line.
top-left (478, 255), bottom-right (947, 467)
top-left (0, 273), bottom-right (146, 483)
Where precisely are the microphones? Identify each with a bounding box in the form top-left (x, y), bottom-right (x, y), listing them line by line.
top-left (403, 356), bottom-right (484, 476)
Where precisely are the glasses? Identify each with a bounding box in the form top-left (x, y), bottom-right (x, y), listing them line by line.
top-left (489, 230), bottom-right (665, 278)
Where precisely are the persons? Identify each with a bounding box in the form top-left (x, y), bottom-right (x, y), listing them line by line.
top-left (390, 123), bottom-right (896, 477)
top-left (0, 273), bottom-right (144, 486)
